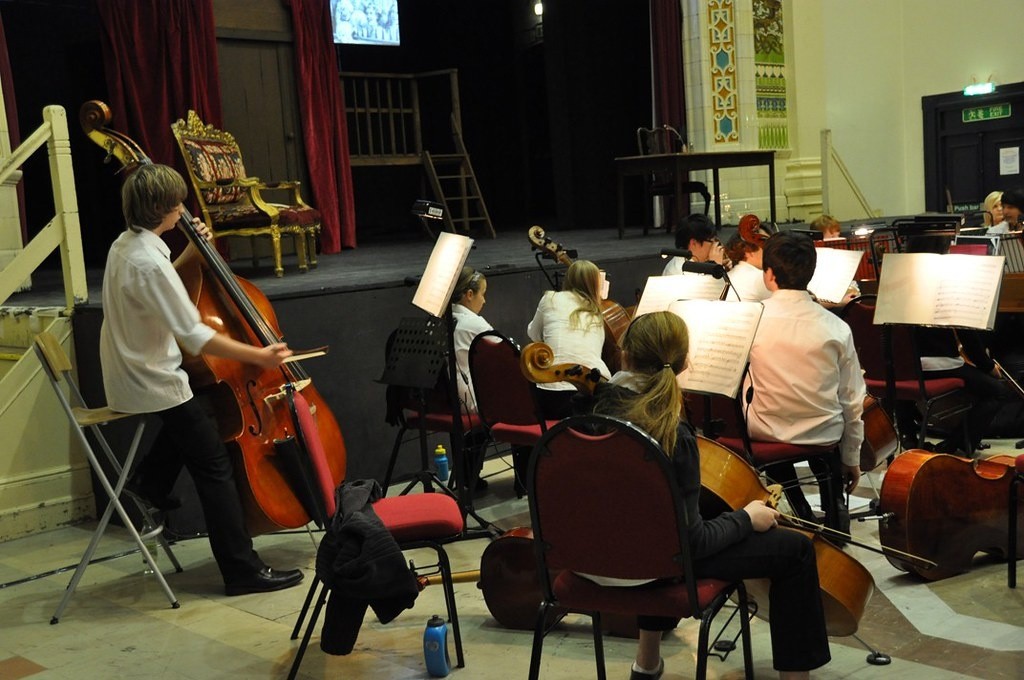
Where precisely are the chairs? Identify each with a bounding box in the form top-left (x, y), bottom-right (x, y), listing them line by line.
top-left (636, 124), bottom-right (712, 236)
top-left (844, 294), bottom-right (977, 461)
top-left (380, 328), bottom-right (481, 499)
top-left (522, 413), bottom-right (755, 680)
top-left (171, 109), bottom-right (323, 279)
top-left (463, 329), bottom-right (562, 503)
top-left (281, 382), bottom-right (466, 680)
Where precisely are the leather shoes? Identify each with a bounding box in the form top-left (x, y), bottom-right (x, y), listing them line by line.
top-left (126, 476), bottom-right (182, 510)
top-left (224, 564), bottom-right (303, 596)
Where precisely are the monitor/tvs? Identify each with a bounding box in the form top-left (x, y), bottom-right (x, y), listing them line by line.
top-left (326, 0), bottom-right (402, 49)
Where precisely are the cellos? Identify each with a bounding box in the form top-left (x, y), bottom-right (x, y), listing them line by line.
top-left (79, 100), bottom-right (346, 555)
top-left (857, 449), bottom-right (1023, 581)
top-left (520, 342), bottom-right (878, 657)
top-left (527, 225), bottom-right (632, 377)
top-left (738, 215), bottom-right (899, 498)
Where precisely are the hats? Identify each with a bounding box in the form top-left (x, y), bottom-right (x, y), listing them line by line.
top-left (1001, 188), bottom-right (1024, 213)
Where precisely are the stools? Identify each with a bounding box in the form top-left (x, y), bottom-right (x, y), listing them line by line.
top-left (29, 330), bottom-right (183, 629)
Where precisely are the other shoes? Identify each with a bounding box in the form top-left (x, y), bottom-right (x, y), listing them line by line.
top-left (792, 499), bottom-right (813, 522)
top-left (821, 499), bottom-right (851, 541)
top-left (629, 657), bottom-right (664, 680)
top-left (463, 474), bottom-right (488, 488)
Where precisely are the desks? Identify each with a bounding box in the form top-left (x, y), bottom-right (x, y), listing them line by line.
top-left (614, 149), bottom-right (776, 241)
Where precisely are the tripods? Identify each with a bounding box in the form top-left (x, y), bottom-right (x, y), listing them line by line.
top-left (381, 317), bottom-right (507, 534)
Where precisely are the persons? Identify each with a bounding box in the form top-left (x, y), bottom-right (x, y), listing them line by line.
top-left (984, 189), bottom-right (1024, 237)
top-left (98, 165), bottom-right (305, 596)
top-left (527, 259), bottom-right (613, 422)
top-left (740, 231), bottom-right (865, 551)
top-left (662, 212), bottom-right (863, 309)
top-left (573, 310), bottom-right (831, 680)
top-left (981, 191), bottom-right (1005, 229)
top-left (427, 266), bottom-right (520, 499)
top-left (906, 210), bottom-right (1001, 450)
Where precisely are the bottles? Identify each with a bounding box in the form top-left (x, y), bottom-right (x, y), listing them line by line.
top-left (424, 614), bottom-right (451, 676)
top-left (434, 444), bottom-right (448, 481)
